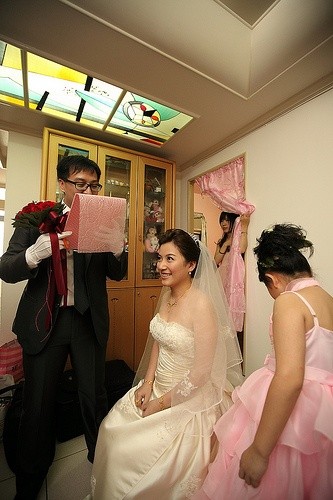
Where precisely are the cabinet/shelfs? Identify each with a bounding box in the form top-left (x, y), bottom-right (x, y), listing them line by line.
top-left (40, 126), bottom-right (177, 373)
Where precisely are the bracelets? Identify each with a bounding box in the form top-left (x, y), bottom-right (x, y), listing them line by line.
top-left (218, 249), bottom-right (226, 255)
top-left (240, 231), bottom-right (248, 235)
top-left (159, 394), bottom-right (168, 411)
top-left (142, 379), bottom-right (154, 385)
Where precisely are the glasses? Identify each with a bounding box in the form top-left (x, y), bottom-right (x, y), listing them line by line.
top-left (61, 177), bottom-right (103, 192)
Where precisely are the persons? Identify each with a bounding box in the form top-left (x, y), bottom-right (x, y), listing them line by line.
top-left (193, 222), bottom-right (333, 500)
top-left (0, 154), bottom-right (128, 500)
top-left (213, 211), bottom-right (251, 269)
top-left (84, 226), bottom-right (245, 500)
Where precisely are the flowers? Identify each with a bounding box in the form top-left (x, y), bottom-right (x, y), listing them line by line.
top-left (12, 200), bottom-right (67, 233)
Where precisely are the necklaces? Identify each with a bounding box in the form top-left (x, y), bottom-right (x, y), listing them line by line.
top-left (165, 283), bottom-right (191, 314)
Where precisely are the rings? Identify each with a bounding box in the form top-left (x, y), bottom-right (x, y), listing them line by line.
top-left (241, 214), bottom-right (244, 216)
top-left (134, 399), bottom-right (142, 403)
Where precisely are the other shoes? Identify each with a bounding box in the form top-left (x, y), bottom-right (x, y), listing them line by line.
top-left (13, 491), bottom-right (38, 500)
top-left (87, 452), bottom-right (94, 463)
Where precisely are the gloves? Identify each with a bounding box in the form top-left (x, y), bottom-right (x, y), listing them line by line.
top-left (25, 230), bottom-right (73, 269)
